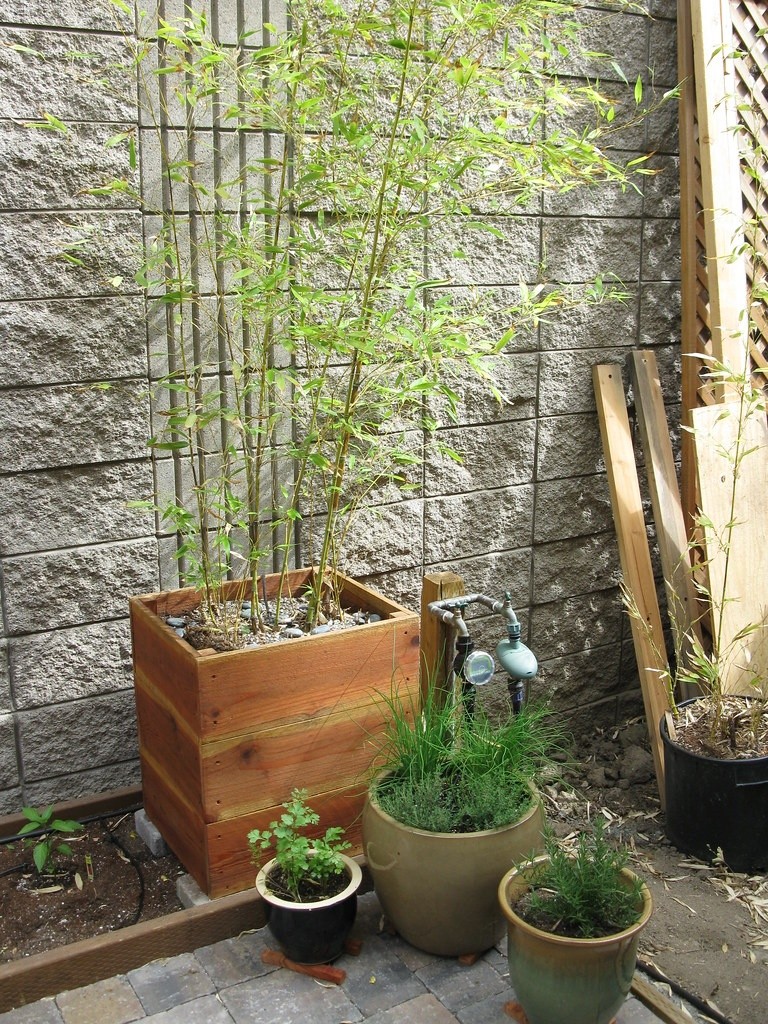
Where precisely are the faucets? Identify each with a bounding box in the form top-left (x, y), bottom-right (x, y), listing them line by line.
top-left (494, 589), bottom-right (519, 625)
top-left (442, 602), bottom-right (471, 637)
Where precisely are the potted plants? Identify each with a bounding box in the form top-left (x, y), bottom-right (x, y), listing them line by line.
top-left (658, 20), bottom-right (768, 876)
top-left (246, 788), bottom-right (361, 964)
top-left (25, 0), bottom-right (700, 904)
top-left (354, 637), bottom-right (576, 955)
top-left (497, 807), bottom-right (653, 1024)
